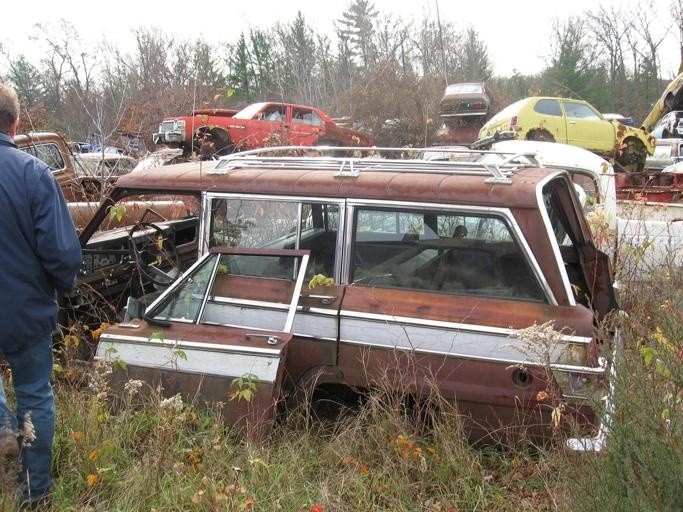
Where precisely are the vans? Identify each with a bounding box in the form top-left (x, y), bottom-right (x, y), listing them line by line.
top-left (50, 146), bottom-right (624, 465)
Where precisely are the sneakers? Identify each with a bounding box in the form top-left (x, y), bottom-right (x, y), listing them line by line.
top-left (0, 434), bottom-right (19, 495)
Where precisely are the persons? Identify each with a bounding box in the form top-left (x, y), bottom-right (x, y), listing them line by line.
top-left (0, 84), bottom-right (82, 509)
